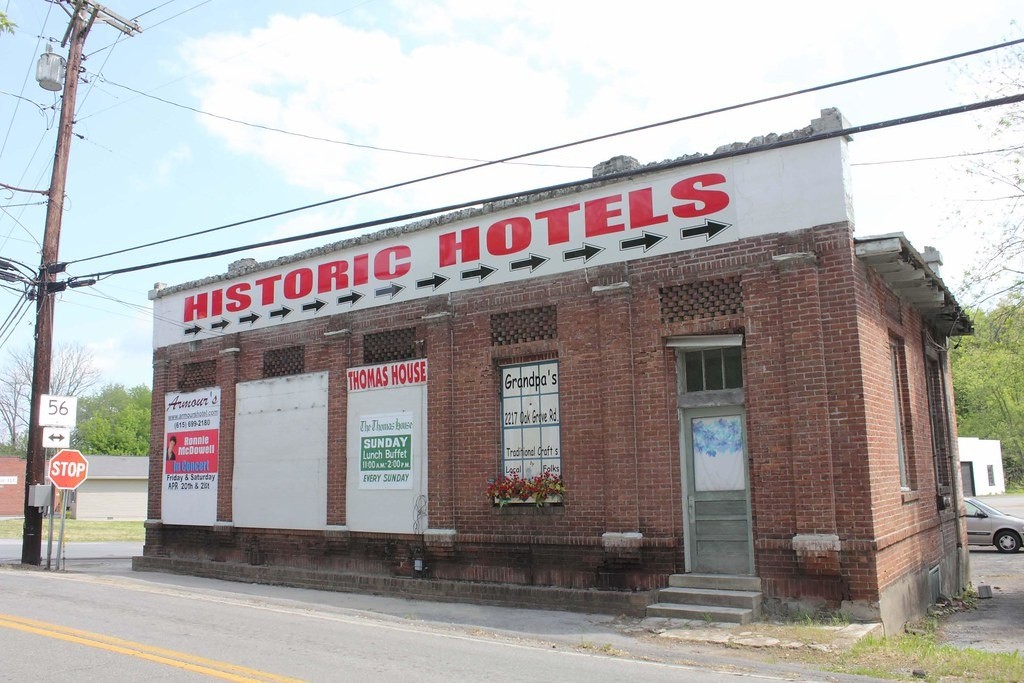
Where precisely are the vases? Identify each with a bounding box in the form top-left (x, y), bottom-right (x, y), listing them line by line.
top-left (495, 494), bottom-right (563, 503)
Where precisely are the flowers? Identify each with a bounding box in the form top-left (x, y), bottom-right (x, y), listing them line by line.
top-left (487, 470), bottom-right (566, 509)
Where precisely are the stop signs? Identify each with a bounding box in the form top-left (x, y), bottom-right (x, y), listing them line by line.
top-left (49, 451), bottom-right (89, 491)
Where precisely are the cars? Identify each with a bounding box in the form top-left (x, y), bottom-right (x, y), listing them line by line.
top-left (962, 497), bottom-right (1024, 552)
